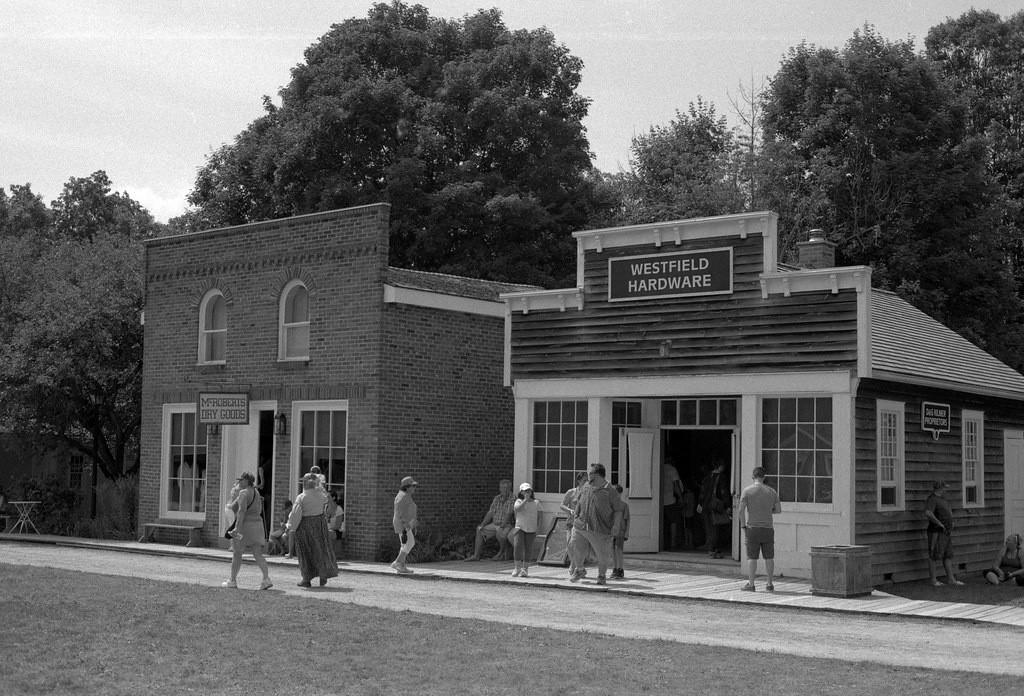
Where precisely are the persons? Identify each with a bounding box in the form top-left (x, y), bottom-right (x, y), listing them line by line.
top-left (222, 470), bottom-right (273, 590)
top-left (664, 449), bottom-right (731, 559)
top-left (389, 477), bottom-right (420, 573)
top-left (270, 465), bottom-right (344, 555)
top-left (559, 463), bottom-right (629, 585)
top-left (464, 479), bottom-right (517, 561)
top-left (284, 474), bottom-right (340, 587)
top-left (739, 467), bottom-right (782, 591)
top-left (511, 483), bottom-right (543, 578)
top-left (983, 534), bottom-right (1024, 587)
top-left (924, 479), bottom-right (964, 586)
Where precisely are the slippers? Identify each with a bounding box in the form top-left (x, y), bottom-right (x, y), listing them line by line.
top-left (930, 581), bottom-right (946, 587)
top-left (948, 580), bottom-right (964, 585)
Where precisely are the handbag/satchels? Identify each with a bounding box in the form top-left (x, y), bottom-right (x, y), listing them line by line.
top-left (225, 521), bottom-right (235, 539)
top-left (709, 495), bottom-right (730, 526)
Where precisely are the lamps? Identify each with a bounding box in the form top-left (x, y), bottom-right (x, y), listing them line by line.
top-left (660, 339), bottom-right (673, 359)
top-left (207, 423), bottom-right (218, 435)
top-left (273, 413), bottom-right (285, 435)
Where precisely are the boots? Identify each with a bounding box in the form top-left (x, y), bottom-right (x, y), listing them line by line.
top-left (521, 562), bottom-right (528, 576)
top-left (512, 560), bottom-right (521, 577)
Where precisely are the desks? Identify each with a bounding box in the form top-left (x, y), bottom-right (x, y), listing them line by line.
top-left (8, 501), bottom-right (42, 536)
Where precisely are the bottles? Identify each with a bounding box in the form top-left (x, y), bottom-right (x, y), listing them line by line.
top-left (228, 532), bottom-right (243, 539)
top-left (401, 529), bottom-right (407, 543)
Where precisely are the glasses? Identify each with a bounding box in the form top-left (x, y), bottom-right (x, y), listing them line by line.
top-left (407, 485), bottom-right (415, 487)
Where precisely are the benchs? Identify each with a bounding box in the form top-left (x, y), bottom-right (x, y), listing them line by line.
top-left (504, 535), bottom-right (546, 561)
top-left (138, 519), bottom-right (205, 547)
top-left (268, 539), bottom-right (345, 559)
top-left (0, 514), bottom-right (19, 533)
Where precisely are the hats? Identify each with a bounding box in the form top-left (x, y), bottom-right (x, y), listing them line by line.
top-left (519, 483), bottom-right (532, 492)
top-left (401, 476), bottom-right (417, 486)
top-left (934, 480), bottom-right (950, 490)
top-left (282, 500), bottom-right (292, 510)
top-left (236, 472), bottom-right (255, 484)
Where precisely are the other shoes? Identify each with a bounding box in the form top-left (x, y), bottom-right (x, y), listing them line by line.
top-left (570, 568), bottom-right (587, 582)
top-left (764, 584), bottom-right (774, 592)
top-left (258, 579), bottom-right (273, 590)
top-left (597, 575), bottom-right (606, 585)
top-left (464, 555), bottom-right (480, 562)
top-left (610, 568), bottom-right (624, 579)
top-left (297, 580), bottom-right (311, 587)
top-left (492, 552), bottom-right (503, 561)
top-left (708, 548), bottom-right (723, 559)
top-left (320, 578), bottom-right (327, 586)
top-left (391, 560), bottom-right (413, 573)
top-left (741, 583), bottom-right (755, 592)
top-left (222, 580), bottom-right (237, 588)
top-left (568, 564), bottom-right (576, 575)
